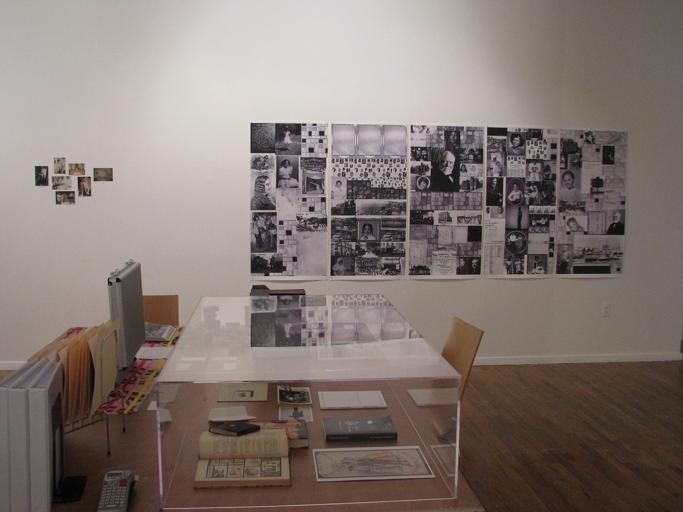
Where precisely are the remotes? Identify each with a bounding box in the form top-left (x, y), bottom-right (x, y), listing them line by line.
top-left (97, 470), bottom-right (132, 512)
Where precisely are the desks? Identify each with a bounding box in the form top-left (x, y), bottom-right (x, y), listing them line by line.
top-left (156, 292), bottom-right (462, 511)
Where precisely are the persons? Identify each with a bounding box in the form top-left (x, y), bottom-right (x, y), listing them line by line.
top-left (247, 123), bottom-right (627, 275)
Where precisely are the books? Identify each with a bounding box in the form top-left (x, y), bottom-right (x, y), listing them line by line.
top-left (317, 390), bottom-right (387, 409)
top-left (320, 414), bottom-right (397, 442)
top-left (246, 419), bottom-right (309, 449)
top-left (209, 422), bottom-right (259, 436)
top-left (190, 428), bottom-right (292, 489)
top-left (407, 387), bottom-right (459, 407)
top-left (143, 324), bottom-right (176, 343)
top-left (277, 385), bottom-right (312, 408)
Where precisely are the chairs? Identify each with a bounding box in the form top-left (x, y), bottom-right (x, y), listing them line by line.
top-left (434, 313), bottom-right (484, 441)
